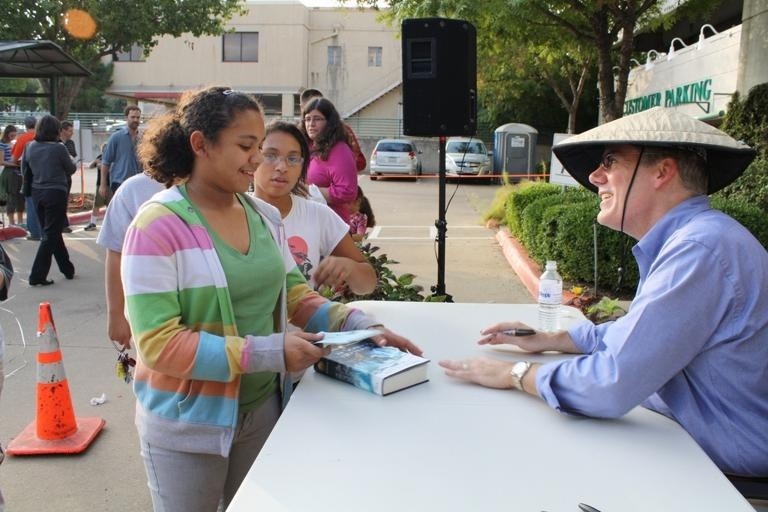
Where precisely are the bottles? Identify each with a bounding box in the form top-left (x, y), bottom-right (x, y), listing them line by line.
top-left (538, 261), bottom-right (563, 333)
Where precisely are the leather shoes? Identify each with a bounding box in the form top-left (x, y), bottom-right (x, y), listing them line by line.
top-left (29, 279), bottom-right (55, 287)
top-left (64, 270), bottom-right (75, 279)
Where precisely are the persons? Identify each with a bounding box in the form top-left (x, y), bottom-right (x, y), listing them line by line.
top-left (1, 124), bottom-right (22, 227)
top-left (20, 115), bottom-right (77, 287)
top-left (291, 180), bottom-right (329, 207)
top-left (300, 96), bottom-right (359, 237)
top-left (0, 242), bottom-right (14, 464)
top-left (440, 105), bottom-right (768, 468)
top-left (118, 84), bottom-right (424, 512)
top-left (93, 170), bottom-right (169, 351)
top-left (297, 88), bottom-right (367, 172)
top-left (97, 105), bottom-right (144, 200)
top-left (10, 115), bottom-right (46, 241)
top-left (58, 119), bottom-right (81, 234)
top-left (83, 142), bottom-right (109, 231)
top-left (250, 120), bottom-right (378, 297)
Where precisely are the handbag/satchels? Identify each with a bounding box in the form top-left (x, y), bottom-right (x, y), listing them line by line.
top-left (8, 144), bottom-right (30, 196)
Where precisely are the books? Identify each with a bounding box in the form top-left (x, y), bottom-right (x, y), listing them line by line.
top-left (312, 338), bottom-right (432, 398)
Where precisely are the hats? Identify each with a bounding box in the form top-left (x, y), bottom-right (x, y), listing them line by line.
top-left (552, 106), bottom-right (756, 194)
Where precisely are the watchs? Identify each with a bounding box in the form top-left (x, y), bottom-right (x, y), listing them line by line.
top-left (509, 359), bottom-right (540, 394)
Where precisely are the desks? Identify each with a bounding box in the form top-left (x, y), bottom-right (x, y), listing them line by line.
top-left (223, 300), bottom-right (757, 512)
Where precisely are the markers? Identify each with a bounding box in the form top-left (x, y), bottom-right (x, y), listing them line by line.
top-left (578, 501), bottom-right (601, 512)
top-left (480, 328), bottom-right (539, 338)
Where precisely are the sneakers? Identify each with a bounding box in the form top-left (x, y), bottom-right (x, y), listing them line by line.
top-left (83, 223), bottom-right (97, 230)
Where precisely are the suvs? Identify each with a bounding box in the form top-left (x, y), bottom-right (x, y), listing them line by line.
top-left (369, 139), bottom-right (423, 182)
top-left (438, 135), bottom-right (494, 183)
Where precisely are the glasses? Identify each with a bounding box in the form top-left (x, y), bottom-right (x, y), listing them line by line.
top-left (303, 116), bottom-right (326, 123)
top-left (262, 151), bottom-right (305, 167)
top-left (599, 151), bottom-right (657, 169)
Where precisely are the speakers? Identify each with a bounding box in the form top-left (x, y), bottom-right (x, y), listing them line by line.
top-left (402, 17), bottom-right (478, 138)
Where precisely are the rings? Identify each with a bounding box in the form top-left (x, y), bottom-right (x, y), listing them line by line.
top-left (462, 362), bottom-right (471, 366)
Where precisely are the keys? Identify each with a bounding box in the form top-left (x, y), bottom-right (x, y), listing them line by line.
top-left (108, 335), bottom-right (137, 385)
